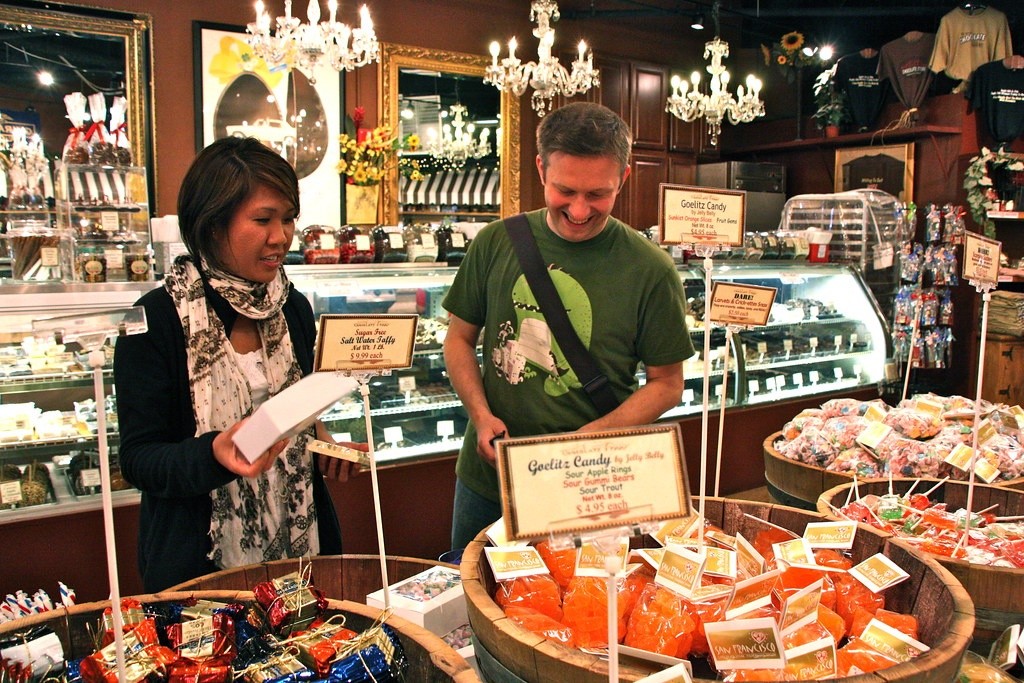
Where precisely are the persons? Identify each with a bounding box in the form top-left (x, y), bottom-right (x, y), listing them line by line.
top-left (115, 136), bottom-right (369, 593)
top-left (442, 102), bottom-right (695, 553)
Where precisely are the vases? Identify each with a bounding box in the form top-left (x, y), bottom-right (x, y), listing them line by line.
top-left (346, 183), bottom-right (380, 224)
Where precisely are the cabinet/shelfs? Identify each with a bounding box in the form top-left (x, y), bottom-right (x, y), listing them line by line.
top-left (944, 153), bottom-right (1024, 407)
top-left (0, 278), bottom-right (170, 599)
top-left (286, 253), bottom-right (895, 557)
top-left (552, 51), bottom-right (718, 231)
top-left (54, 162), bottom-right (155, 283)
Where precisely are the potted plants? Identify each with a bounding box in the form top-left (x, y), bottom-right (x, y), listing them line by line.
top-left (811, 70), bottom-right (844, 138)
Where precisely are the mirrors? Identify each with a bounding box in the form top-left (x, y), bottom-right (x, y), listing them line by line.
top-left (376, 42), bottom-right (521, 225)
top-left (0, 0), bottom-right (163, 281)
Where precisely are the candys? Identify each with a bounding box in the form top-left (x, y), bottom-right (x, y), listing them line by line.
top-left (504, 522), bottom-right (918, 675)
top-left (777, 396), bottom-right (1024, 568)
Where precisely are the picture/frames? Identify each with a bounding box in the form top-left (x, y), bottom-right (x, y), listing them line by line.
top-left (191, 18), bottom-right (347, 250)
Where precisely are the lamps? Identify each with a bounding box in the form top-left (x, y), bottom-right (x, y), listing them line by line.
top-left (481, 0), bottom-right (600, 117)
top-left (247, 0), bottom-right (381, 86)
top-left (690, 1), bottom-right (705, 31)
top-left (401, 100), bottom-right (416, 119)
top-left (427, 75), bottom-right (492, 172)
top-left (665, 0), bottom-right (766, 145)
